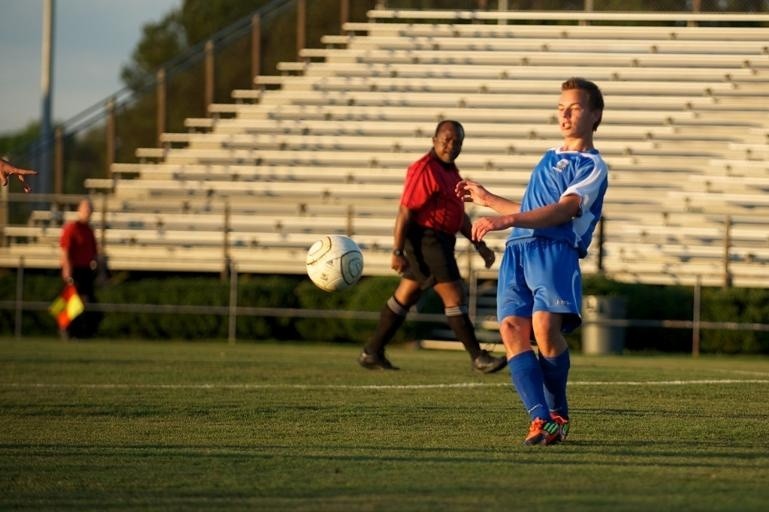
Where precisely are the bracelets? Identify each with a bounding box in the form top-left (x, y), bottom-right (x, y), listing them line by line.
top-left (475, 238), bottom-right (486, 251)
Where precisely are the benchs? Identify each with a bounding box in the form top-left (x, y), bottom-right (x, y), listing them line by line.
top-left (0, 10), bottom-right (769, 289)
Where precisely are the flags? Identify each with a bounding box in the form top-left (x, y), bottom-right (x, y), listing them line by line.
top-left (46, 280), bottom-right (87, 332)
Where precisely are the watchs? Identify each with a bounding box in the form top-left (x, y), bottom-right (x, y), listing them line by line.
top-left (393, 247), bottom-right (404, 256)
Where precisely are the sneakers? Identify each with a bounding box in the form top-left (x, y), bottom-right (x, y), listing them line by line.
top-left (524, 416), bottom-right (569, 445)
top-left (359, 349), bottom-right (400, 371)
top-left (472, 350), bottom-right (509, 375)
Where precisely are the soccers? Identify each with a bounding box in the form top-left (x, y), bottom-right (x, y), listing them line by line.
top-left (305, 235), bottom-right (365, 294)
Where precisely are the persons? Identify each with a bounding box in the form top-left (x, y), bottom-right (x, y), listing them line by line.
top-left (357, 119), bottom-right (508, 375)
top-left (0, 159), bottom-right (40, 194)
top-left (454, 77), bottom-right (609, 443)
top-left (58, 196), bottom-right (106, 341)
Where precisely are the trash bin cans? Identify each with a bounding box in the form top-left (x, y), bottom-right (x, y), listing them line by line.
top-left (581, 294), bottom-right (628, 356)
